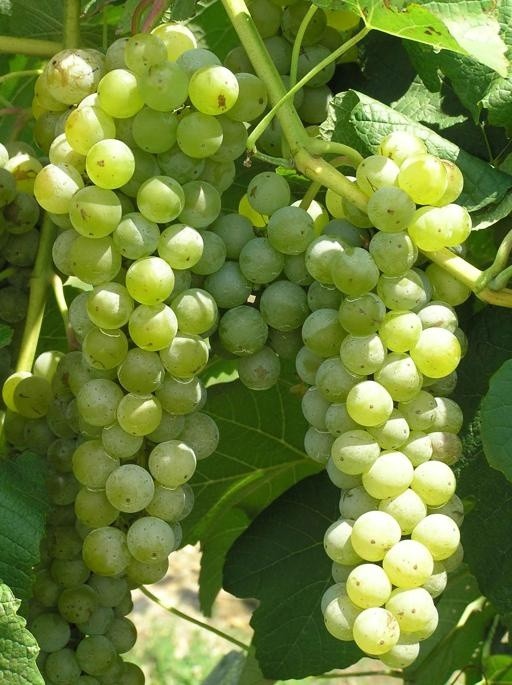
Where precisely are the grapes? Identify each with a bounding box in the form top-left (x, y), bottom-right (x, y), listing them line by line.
top-left (0, 1), bottom-right (473, 685)
